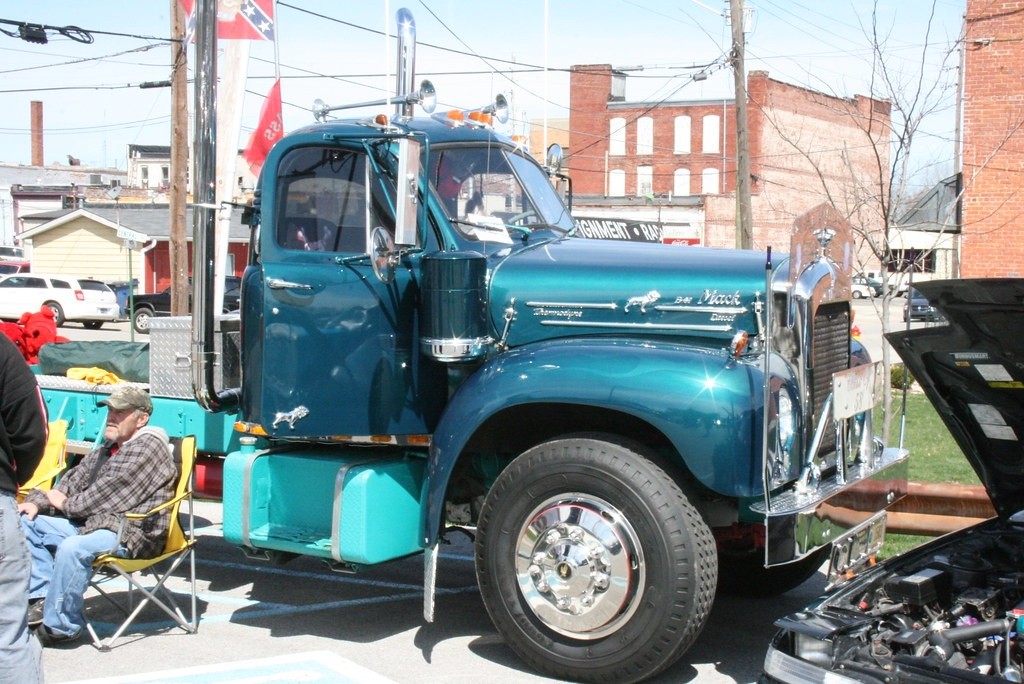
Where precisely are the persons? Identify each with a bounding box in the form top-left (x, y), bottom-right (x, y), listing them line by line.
top-left (18, 386), bottom-right (178, 647)
top-left (0, 330), bottom-right (50, 684)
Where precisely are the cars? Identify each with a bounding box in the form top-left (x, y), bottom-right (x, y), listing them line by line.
top-left (125, 275), bottom-right (243, 335)
top-left (903, 288), bottom-right (944, 323)
top-left (850, 269), bottom-right (909, 299)
top-left (758, 276), bottom-right (1023, 684)
top-left (0, 246), bottom-right (120, 330)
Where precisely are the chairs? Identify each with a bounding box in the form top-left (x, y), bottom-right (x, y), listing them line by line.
top-left (18, 419), bottom-right (68, 505)
top-left (82, 434), bottom-right (200, 652)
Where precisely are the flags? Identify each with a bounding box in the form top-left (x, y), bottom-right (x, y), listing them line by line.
top-left (243, 79), bottom-right (284, 178)
top-left (180, 0), bottom-right (275, 41)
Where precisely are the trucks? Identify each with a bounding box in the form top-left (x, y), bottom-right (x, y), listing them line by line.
top-left (27, 78), bottom-right (917, 684)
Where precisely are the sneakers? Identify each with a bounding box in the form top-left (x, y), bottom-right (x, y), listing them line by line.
top-left (28, 598), bottom-right (44, 625)
top-left (31, 623), bottom-right (82, 646)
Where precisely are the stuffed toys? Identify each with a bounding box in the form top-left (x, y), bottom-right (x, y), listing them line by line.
top-left (0, 305), bottom-right (70, 365)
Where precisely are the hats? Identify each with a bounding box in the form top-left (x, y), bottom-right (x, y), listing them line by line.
top-left (96, 386), bottom-right (153, 417)
